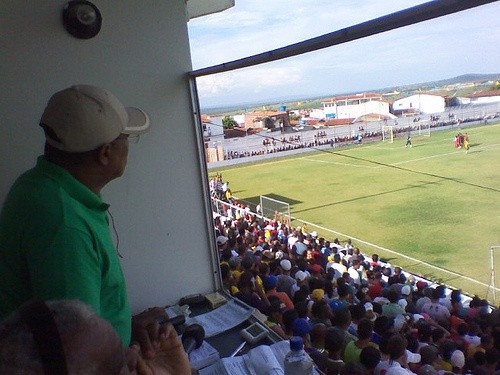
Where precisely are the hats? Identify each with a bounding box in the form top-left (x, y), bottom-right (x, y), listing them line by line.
top-left (264, 275), bottom-right (277, 288)
top-left (399, 299), bottom-right (407, 306)
top-left (451, 350), bottom-right (465, 367)
top-left (295, 270), bottom-right (310, 281)
top-left (406, 350), bottom-right (421, 364)
top-left (280, 259), bottom-right (291, 270)
top-left (313, 289), bottom-right (324, 300)
top-left (401, 285), bottom-right (411, 295)
top-left (39, 84), bottom-right (148, 151)
top-left (365, 302), bottom-right (373, 311)
top-left (293, 318), bottom-right (313, 334)
top-left (272, 301), bottom-right (287, 313)
top-left (229, 255), bottom-right (242, 267)
top-left (217, 236), bottom-right (229, 244)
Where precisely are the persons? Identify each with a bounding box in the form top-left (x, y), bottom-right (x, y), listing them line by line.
top-left (223, 110), bottom-right (500, 159)
top-left (0, 84), bottom-right (192, 375)
top-left (209, 175), bottom-right (263, 220)
top-left (208, 209), bottom-right (500, 375)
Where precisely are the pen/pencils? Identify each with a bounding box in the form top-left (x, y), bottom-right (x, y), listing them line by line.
top-left (229, 341), bottom-right (247, 358)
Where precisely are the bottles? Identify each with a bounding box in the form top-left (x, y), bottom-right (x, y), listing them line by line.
top-left (284, 336), bottom-right (313, 375)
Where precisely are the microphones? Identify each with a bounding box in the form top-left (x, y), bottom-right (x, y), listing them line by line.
top-left (182, 324), bottom-right (205, 355)
